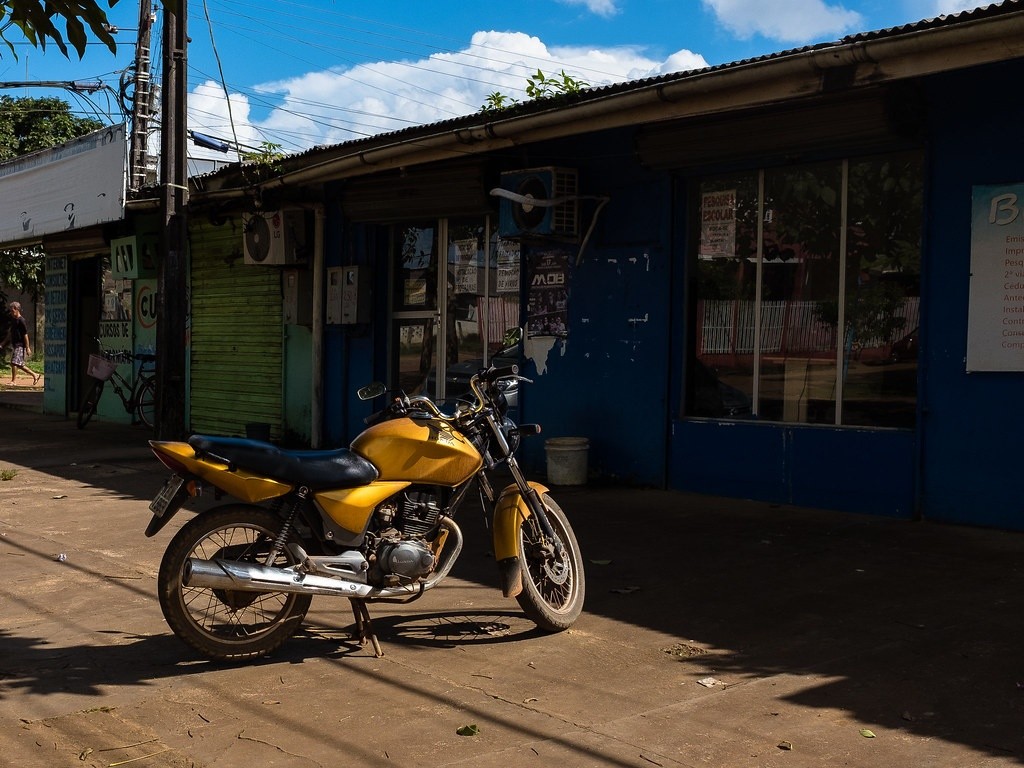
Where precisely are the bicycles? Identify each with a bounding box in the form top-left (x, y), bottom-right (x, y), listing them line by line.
top-left (77, 330), bottom-right (186, 431)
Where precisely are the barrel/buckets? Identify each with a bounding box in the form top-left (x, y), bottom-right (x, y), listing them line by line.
top-left (546, 437), bottom-right (590, 485)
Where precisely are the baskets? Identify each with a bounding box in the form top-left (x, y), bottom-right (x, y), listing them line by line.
top-left (87, 354), bottom-right (118, 381)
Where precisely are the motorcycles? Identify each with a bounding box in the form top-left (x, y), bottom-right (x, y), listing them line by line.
top-left (145, 327), bottom-right (585, 664)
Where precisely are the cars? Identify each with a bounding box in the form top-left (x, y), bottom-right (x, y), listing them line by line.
top-left (408, 345), bottom-right (518, 427)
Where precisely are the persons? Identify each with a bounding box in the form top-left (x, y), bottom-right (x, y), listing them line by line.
top-left (0, 301), bottom-right (41, 385)
top-left (528, 289), bottom-right (569, 336)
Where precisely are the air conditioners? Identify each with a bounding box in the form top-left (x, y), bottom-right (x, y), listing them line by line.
top-left (242, 211), bottom-right (308, 264)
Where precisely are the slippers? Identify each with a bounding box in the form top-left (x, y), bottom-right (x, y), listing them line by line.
top-left (6, 382), bottom-right (16, 386)
top-left (33, 374), bottom-right (41, 385)
